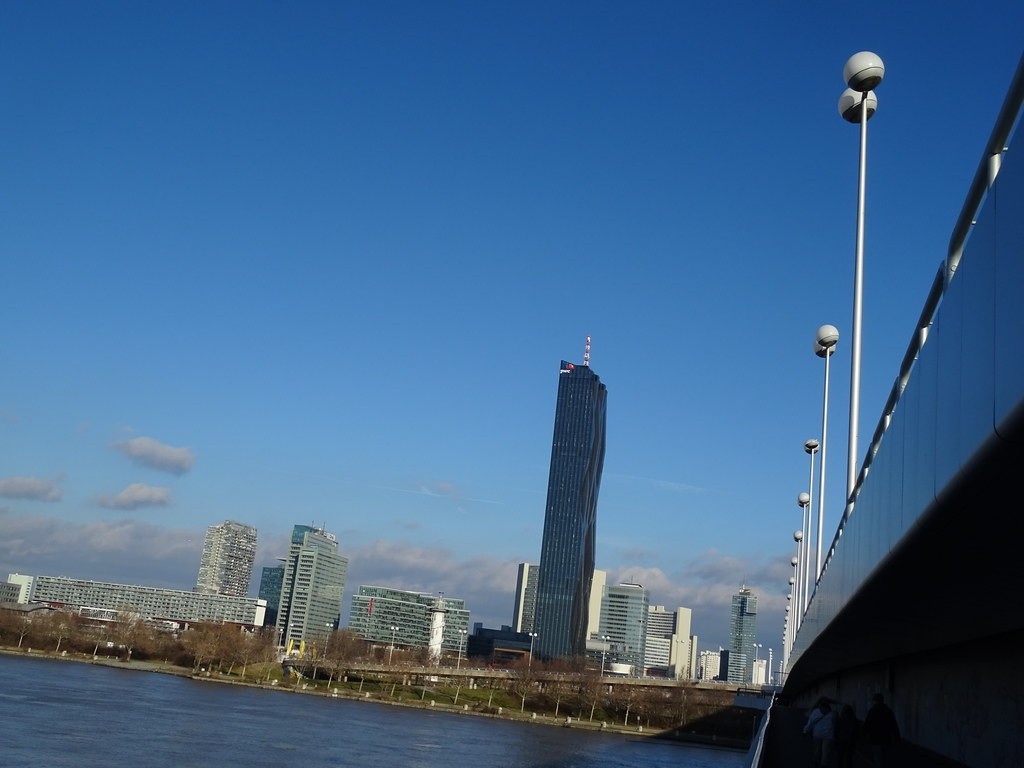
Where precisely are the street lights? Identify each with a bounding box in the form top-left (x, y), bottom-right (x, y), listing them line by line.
top-left (387, 625), bottom-right (399, 665)
top-left (752, 642), bottom-right (764, 686)
top-left (811, 324), bottom-right (842, 585)
top-left (457, 628), bottom-right (467, 670)
top-left (780, 436), bottom-right (820, 677)
top-left (838, 50), bottom-right (887, 503)
top-left (599, 636), bottom-right (612, 678)
top-left (321, 622), bottom-right (334, 663)
top-left (527, 631), bottom-right (538, 675)
